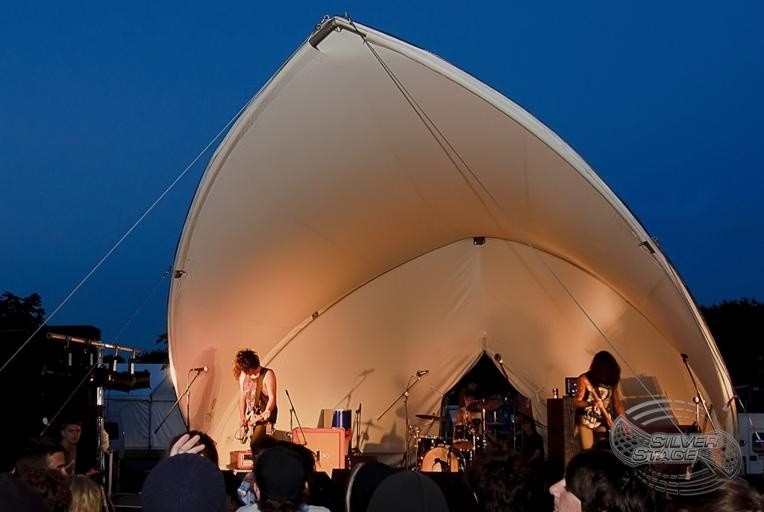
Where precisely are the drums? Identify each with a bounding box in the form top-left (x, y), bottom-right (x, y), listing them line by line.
top-left (332, 409), bottom-right (351, 431)
top-left (419, 444), bottom-right (466, 473)
top-left (416, 436), bottom-right (453, 471)
top-left (452, 424), bottom-right (473, 451)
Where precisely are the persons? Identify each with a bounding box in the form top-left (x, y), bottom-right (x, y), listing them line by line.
top-left (233, 348), bottom-right (278, 436)
top-left (0, 416), bottom-right (764, 511)
top-left (573, 350), bottom-right (631, 450)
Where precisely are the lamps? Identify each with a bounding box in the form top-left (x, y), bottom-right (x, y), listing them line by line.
top-left (310, 17), bottom-right (366, 60)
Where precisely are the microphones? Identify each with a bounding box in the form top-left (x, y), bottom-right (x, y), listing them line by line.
top-left (413, 371), bottom-right (429, 377)
top-left (680, 353), bottom-right (688, 358)
top-left (190, 366), bottom-right (209, 372)
top-left (494, 354), bottom-right (503, 365)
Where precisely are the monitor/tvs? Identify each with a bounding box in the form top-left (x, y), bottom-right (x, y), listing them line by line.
top-left (566, 378), bottom-right (579, 394)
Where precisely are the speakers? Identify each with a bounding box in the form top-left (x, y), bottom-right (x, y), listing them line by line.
top-left (332, 468), bottom-right (481, 512)
top-left (143, 469), bottom-right (235, 499)
top-left (236, 471), bottom-right (331, 506)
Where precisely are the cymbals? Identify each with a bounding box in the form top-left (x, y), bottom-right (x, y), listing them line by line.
top-left (467, 399), bottom-right (503, 412)
top-left (416, 414), bottom-right (450, 422)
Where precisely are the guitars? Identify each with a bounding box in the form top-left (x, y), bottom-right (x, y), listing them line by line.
top-left (239, 400), bottom-right (261, 444)
top-left (592, 388), bottom-right (633, 438)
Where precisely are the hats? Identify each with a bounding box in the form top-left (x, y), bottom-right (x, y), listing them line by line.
top-left (144, 453), bottom-right (226, 512)
top-left (346, 461), bottom-right (449, 512)
